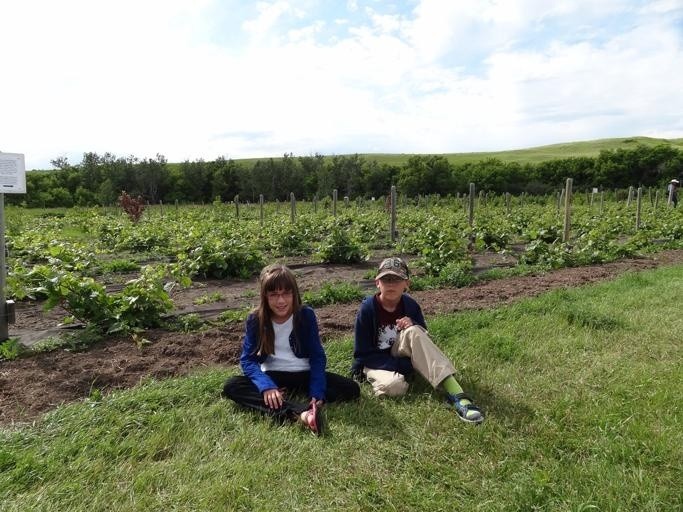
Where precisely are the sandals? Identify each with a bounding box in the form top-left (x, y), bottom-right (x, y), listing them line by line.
top-left (446, 393), bottom-right (485, 424)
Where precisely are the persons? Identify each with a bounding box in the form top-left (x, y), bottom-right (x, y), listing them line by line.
top-left (222, 264), bottom-right (361, 433)
top-left (352, 257), bottom-right (483, 424)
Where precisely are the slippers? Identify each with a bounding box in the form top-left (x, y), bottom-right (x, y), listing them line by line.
top-left (306, 401), bottom-right (320, 437)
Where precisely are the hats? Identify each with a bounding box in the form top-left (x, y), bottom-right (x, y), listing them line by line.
top-left (375, 257), bottom-right (409, 281)
